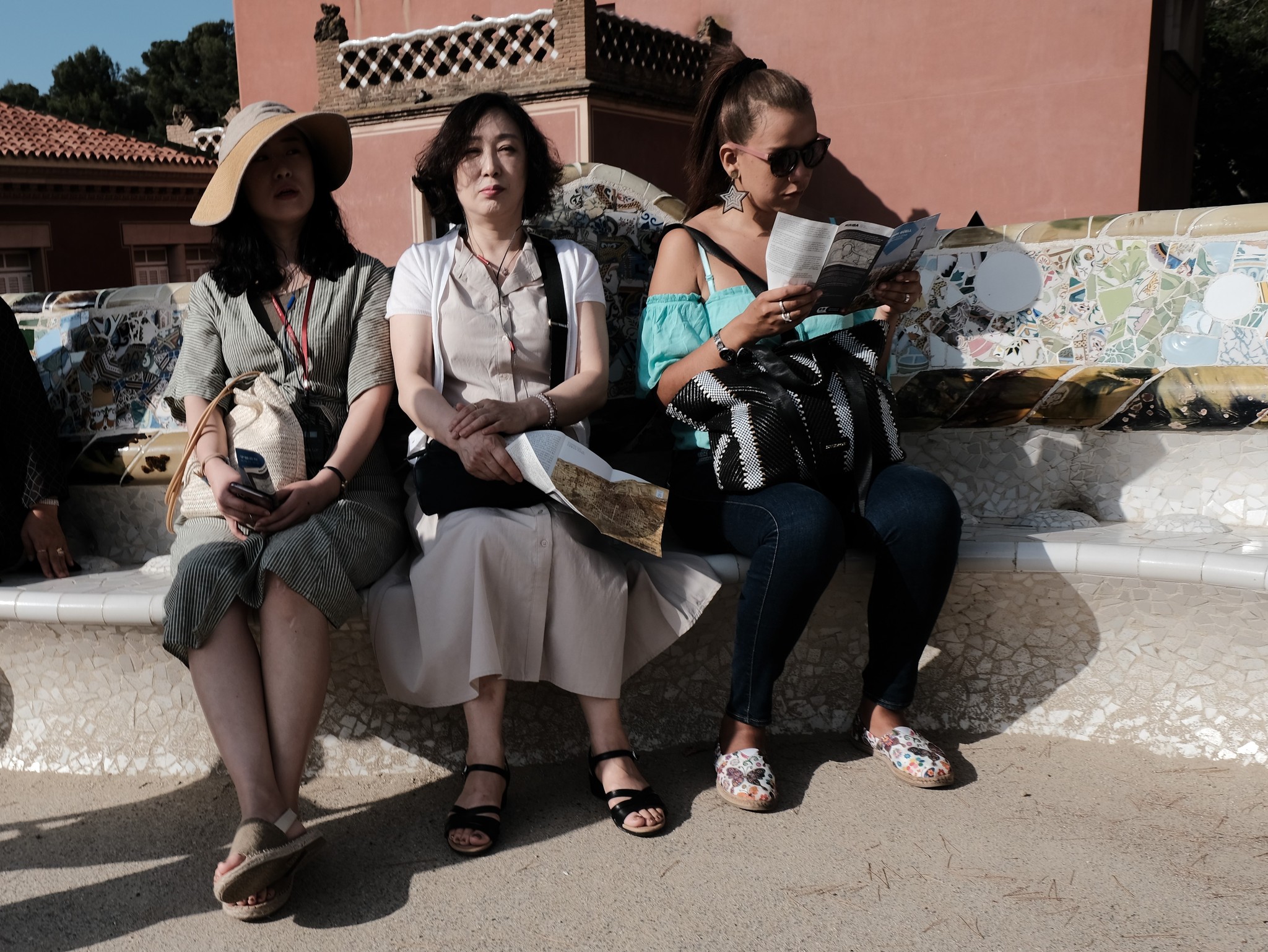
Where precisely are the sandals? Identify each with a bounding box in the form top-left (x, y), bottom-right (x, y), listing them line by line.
top-left (587, 741), bottom-right (666, 835)
top-left (444, 751), bottom-right (513, 853)
top-left (212, 807), bottom-right (325, 920)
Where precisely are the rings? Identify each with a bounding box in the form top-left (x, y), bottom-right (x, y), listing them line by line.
top-left (36, 549), bottom-right (46, 552)
top-left (471, 403), bottom-right (478, 409)
top-left (782, 312), bottom-right (792, 324)
top-left (779, 300), bottom-right (785, 313)
top-left (902, 293), bottom-right (910, 303)
top-left (57, 548), bottom-right (64, 557)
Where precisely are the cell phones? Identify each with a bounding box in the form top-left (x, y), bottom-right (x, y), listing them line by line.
top-left (228, 481), bottom-right (274, 514)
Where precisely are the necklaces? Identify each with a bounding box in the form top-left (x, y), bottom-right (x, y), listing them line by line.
top-left (501, 241), bottom-right (522, 275)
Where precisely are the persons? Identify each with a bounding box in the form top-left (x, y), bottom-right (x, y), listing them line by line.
top-left (639, 42), bottom-right (964, 811)
top-left (359, 93), bottom-right (721, 857)
top-left (0, 297), bottom-right (74, 578)
top-left (159, 101), bottom-right (407, 916)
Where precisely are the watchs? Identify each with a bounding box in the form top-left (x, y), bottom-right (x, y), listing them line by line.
top-left (319, 466), bottom-right (349, 497)
top-left (714, 328), bottom-right (735, 365)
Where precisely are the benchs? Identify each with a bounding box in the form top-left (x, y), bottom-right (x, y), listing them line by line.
top-left (0, 411), bottom-right (1268, 811)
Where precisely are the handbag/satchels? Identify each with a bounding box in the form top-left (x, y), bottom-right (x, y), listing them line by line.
top-left (410, 439), bottom-right (544, 516)
top-left (163, 372), bottom-right (306, 534)
top-left (664, 317), bottom-right (908, 493)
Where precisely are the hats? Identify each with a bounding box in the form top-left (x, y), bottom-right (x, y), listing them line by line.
top-left (188, 100), bottom-right (353, 226)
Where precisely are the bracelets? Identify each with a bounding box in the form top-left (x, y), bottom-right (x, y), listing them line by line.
top-left (38, 499), bottom-right (59, 506)
top-left (529, 393), bottom-right (558, 427)
top-left (200, 453), bottom-right (231, 477)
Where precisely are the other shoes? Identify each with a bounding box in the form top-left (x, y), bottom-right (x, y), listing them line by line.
top-left (849, 712), bottom-right (954, 787)
top-left (708, 732), bottom-right (777, 810)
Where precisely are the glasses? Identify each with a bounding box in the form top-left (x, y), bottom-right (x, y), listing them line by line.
top-left (729, 132), bottom-right (831, 179)
